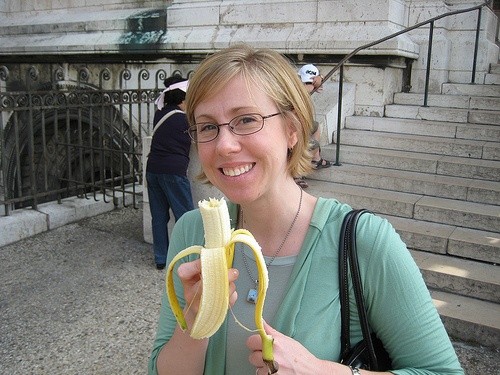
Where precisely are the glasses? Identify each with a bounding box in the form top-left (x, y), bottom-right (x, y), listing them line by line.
top-left (185, 112), bottom-right (284, 142)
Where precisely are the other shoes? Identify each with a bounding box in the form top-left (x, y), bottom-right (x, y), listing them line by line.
top-left (157, 263), bottom-right (166, 270)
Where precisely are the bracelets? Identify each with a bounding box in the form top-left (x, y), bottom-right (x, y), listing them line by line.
top-left (349, 365), bottom-right (360, 375)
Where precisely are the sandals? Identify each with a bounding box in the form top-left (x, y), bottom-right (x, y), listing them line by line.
top-left (311, 157), bottom-right (333, 170)
top-left (293, 177), bottom-right (308, 188)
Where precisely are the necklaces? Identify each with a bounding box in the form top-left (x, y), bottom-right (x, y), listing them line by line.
top-left (240, 183), bottom-right (303, 305)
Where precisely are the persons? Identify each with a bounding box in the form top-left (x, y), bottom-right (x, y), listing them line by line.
top-left (147, 43), bottom-right (464, 375)
top-left (146, 74), bottom-right (196, 269)
top-left (284, 64), bottom-right (333, 188)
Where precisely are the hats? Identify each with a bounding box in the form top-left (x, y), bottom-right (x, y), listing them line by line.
top-left (156, 73), bottom-right (189, 110)
top-left (298, 63), bottom-right (320, 84)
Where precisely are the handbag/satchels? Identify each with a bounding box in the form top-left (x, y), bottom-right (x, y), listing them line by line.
top-left (335, 208), bottom-right (396, 372)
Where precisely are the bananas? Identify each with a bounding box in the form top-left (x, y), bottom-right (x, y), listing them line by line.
top-left (165, 196), bottom-right (275, 373)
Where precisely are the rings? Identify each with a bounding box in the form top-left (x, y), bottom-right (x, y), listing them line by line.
top-left (255, 366), bottom-right (261, 375)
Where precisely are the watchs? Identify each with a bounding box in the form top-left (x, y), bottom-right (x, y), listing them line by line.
top-left (318, 74), bottom-right (325, 81)
